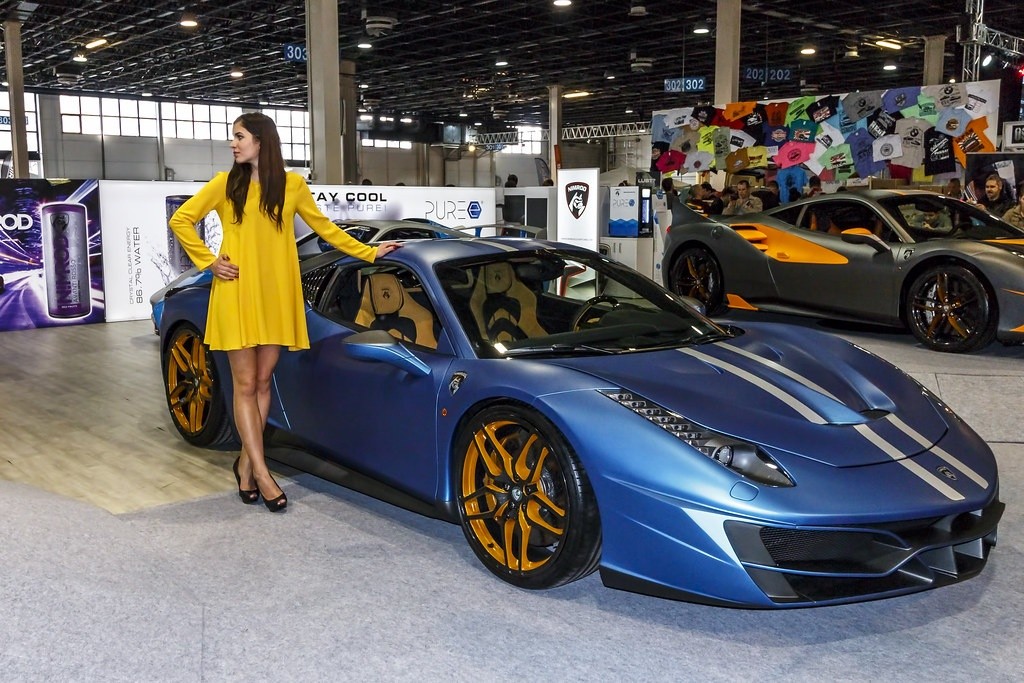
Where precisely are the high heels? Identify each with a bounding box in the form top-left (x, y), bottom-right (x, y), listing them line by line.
top-left (258, 474), bottom-right (288, 512)
top-left (233, 456), bottom-right (259, 504)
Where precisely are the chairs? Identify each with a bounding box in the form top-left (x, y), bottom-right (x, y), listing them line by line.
top-left (869, 209), bottom-right (904, 241)
top-left (345, 273), bottom-right (440, 351)
top-left (462, 260), bottom-right (549, 345)
top-left (807, 211), bottom-right (843, 238)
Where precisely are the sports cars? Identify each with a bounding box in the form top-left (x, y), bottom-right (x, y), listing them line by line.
top-left (149, 216), bottom-right (1006, 609)
top-left (150, 220), bottom-right (586, 335)
top-left (660, 189), bottom-right (1024, 352)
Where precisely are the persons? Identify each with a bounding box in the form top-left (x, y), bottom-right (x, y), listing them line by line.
top-left (912, 204), bottom-right (951, 232)
top-left (167, 113), bottom-right (405, 513)
top-left (966, 174), bottom-right (1015, 218)
top-left (361, 172), bottom-right (553, 187)
top-left (1002, 180), bottom-right (1024, 231)
top-left (947, 178), bottom-right (963, 199)
top-left (661, 176), bottom-right (848, 215)
top-left (721, 180), bottom-right (763, 216)
